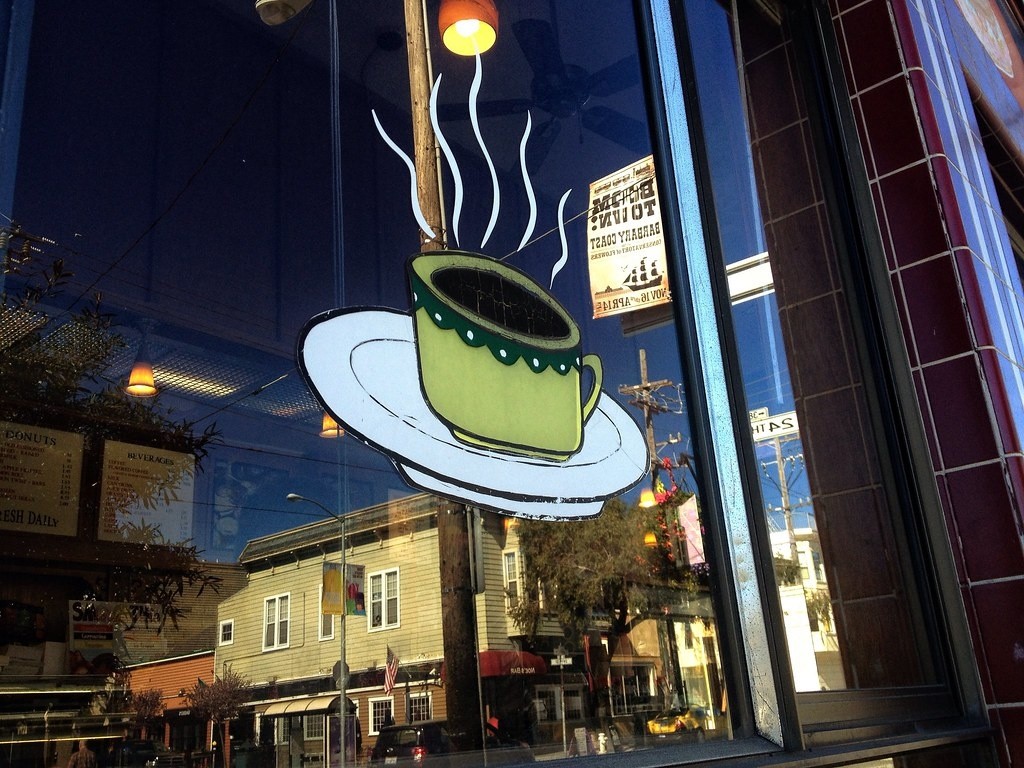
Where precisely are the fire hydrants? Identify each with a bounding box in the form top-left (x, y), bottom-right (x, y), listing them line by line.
top-left (597, 732), bottom-right (608, 756)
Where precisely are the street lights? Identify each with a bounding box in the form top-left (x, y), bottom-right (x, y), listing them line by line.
top-left (285, 493), bottom-right (346, 768)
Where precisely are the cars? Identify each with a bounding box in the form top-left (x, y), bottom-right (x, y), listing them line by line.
top-left (648, 706), bottom-right (717, 744)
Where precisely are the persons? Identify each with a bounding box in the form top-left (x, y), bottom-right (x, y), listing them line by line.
top-left (67, 739), bottom-right (99, 768)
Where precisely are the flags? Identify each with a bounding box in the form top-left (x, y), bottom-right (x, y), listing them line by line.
top-left (383, 646), bottom-right (399, 697)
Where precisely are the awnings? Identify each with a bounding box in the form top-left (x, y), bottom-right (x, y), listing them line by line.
top-left (440, 651), bottom-right (547, 682)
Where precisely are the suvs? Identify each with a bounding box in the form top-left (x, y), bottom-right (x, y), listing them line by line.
top-left (110, 738), bottom-right (188, 768)
top-left (369, 717), bottom-right (536, 768)
